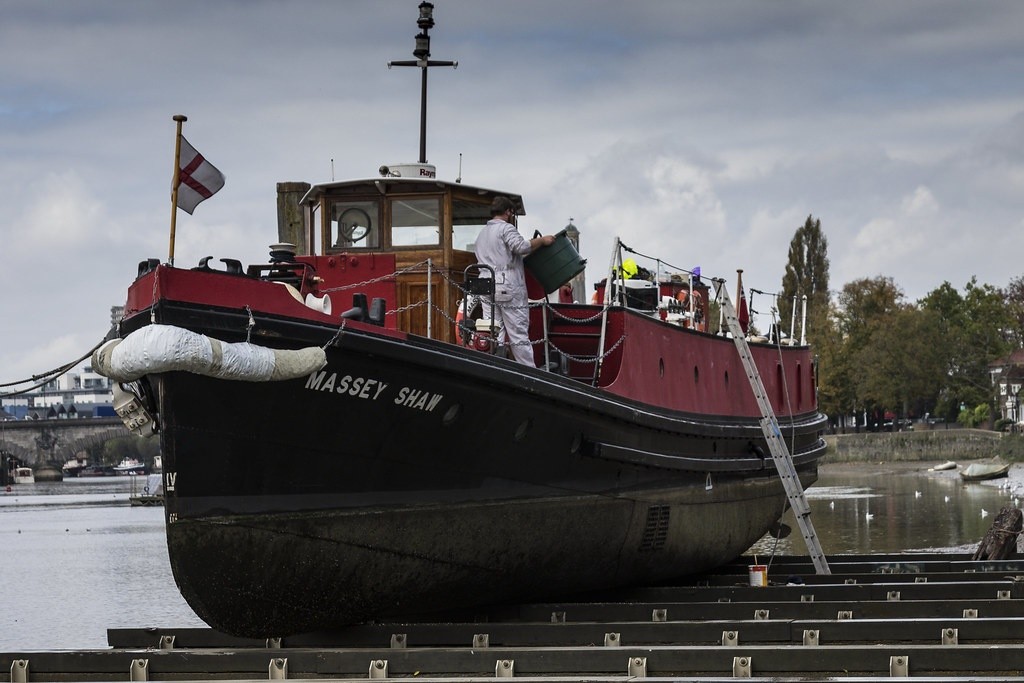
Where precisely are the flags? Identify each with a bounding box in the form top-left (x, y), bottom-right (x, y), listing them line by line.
top-left (738, 280), bottom-right (749, 334)
top-left (170, 134), bottom-right (226, 215)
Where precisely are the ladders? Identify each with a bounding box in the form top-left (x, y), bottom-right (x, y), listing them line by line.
top-left (705, 277), bottom-right (834, 575)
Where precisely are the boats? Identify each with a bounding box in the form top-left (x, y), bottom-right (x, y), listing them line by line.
top-left (62, 456), bottom-right (162, 486)
top-left (89, 1), bottom-right (829, 638)
top-left (959, 461), bottom-right (1009, 481)
top-left (128, 465), bottom-right (163, 507)
top-left (15, 468), bottom-right (35, 484)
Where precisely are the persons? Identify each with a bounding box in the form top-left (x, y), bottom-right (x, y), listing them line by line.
top-left (475, 197), bottom-right (556, 368)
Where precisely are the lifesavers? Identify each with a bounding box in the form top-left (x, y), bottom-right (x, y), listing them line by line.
top-left (678, 289), bottom-right (706, 332)
top-left (455, 290), bottom-right (486, 351)
top-left (592, 290), bottom-right (598, 304)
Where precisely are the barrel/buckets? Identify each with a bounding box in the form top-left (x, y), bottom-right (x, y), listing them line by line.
top-left (523, 229), bottom-right (587, 294)
top-left (748, 565), bottom-right (767, 587)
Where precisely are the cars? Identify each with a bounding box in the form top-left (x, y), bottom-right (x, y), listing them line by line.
top-left (870, 418), bottom-right (912, 432)
